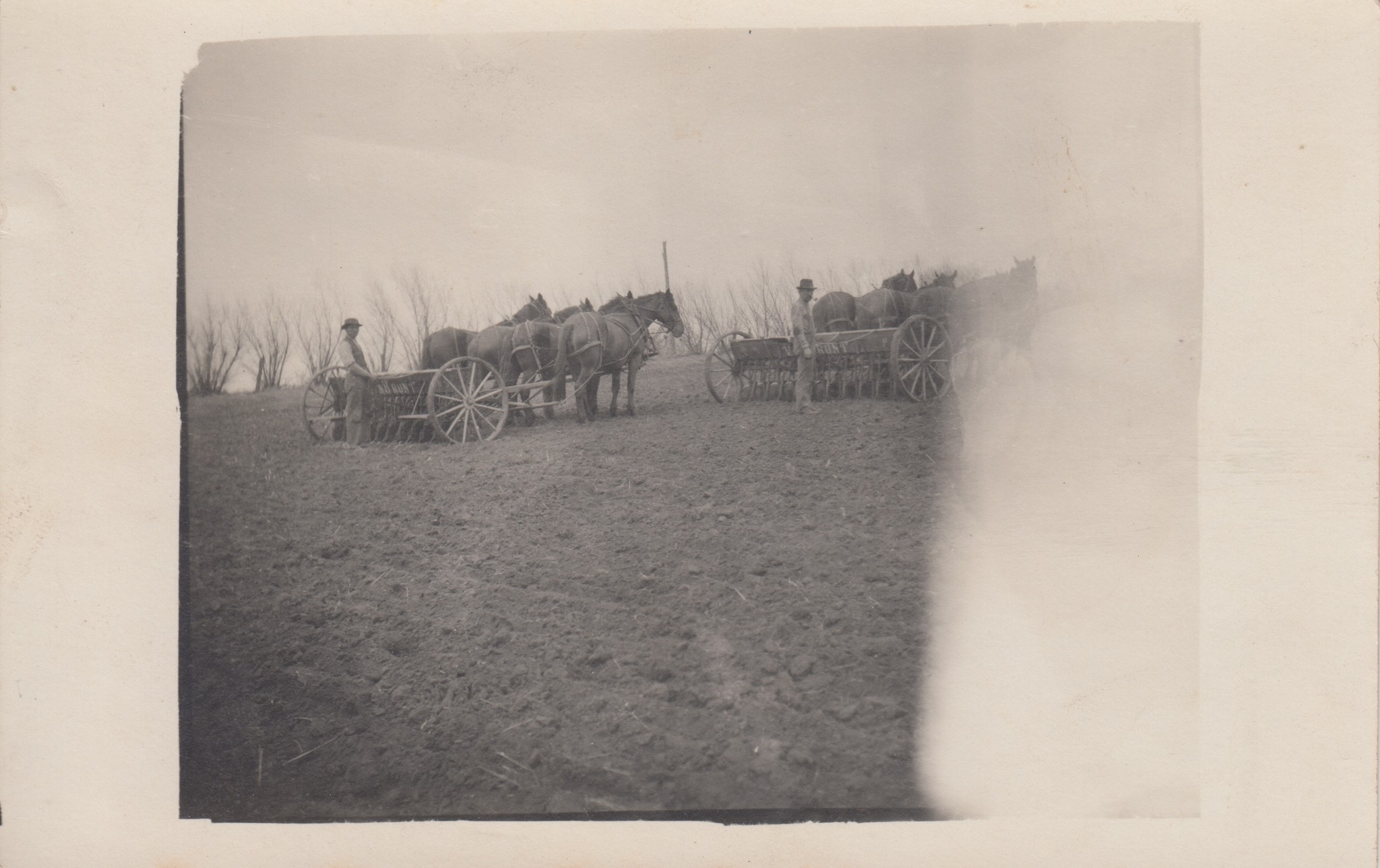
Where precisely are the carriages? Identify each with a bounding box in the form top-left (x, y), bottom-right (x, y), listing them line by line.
top-left (302, 287), bottom-right (687, 444)
top-left (703, 254), bottom-right (1041, 406)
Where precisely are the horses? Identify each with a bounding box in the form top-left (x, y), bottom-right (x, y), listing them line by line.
top-left (808, 253), bottom-right (1041, 400)
top-left (418, 286), bottom-right (688, 427)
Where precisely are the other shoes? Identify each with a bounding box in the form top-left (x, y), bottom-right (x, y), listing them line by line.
top-left (799, 409), bottom-right (820, 415)
top-left (812, 407), bottom-right (821, 411)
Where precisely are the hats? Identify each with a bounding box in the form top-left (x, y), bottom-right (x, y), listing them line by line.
top-left (341, 318), bottom-right (363, 329)
top-left (797, 278), bottom-right (817, 291)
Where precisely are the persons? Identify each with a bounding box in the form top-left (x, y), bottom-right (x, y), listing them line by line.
top-left (791, 279), bottom-right (822, 414)
top-left (337, 318), bottom-right (377, 444)
